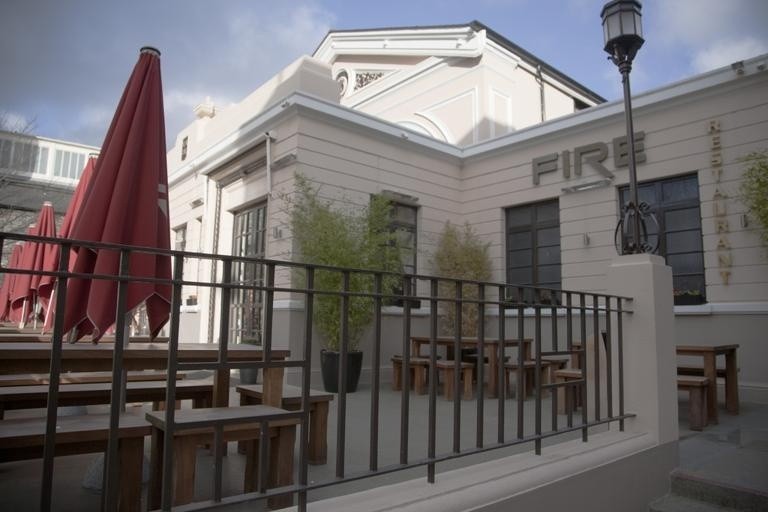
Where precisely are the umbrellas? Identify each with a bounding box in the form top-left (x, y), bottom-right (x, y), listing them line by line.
top-left (52, 47), bottom-right (173, 412)
top-left (0, 153), bottom-right (97, 334)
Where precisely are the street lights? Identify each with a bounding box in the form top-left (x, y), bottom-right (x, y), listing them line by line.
top-left (597, 0), bottom-right (662, 256)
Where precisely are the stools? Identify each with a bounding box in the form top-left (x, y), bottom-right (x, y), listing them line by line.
top-left (436, 360), bottom-right (473, 400)
top-left (390, 355), bottom-right (430, 395)
top-left (467, 353), bottom-right (510, 384)
top-left (532, 358), bottom-right (568, 391)
top-left (504, 361), bottom-right (550, 399)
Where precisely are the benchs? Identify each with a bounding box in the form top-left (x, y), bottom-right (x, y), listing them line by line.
top-left (556, 369), bottom-right (711, 432)
top-left (677, 366), bottom-right (740, 409)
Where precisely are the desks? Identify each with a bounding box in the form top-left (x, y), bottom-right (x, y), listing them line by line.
top-left (409, 334), bottom-right (534, 398)
top-left (0, 316), bottom-right (334, 511)
top-left (570, 338), bottom-right (739, 425)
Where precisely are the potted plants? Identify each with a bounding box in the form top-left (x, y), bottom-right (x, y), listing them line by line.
top-left (274, 166), bottom-right (403, 393)
top-left (421, 216), bottom-right (494, 380)
